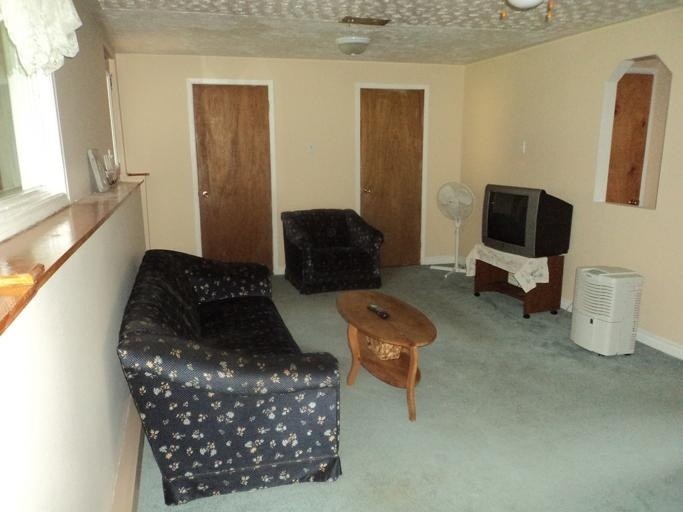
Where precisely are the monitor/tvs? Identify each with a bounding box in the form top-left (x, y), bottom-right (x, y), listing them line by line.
top-left (482, 184), bottom-right (573, 257)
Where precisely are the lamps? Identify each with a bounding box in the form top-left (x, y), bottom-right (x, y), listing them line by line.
top-left (333, 35), bottom-right (370, 58)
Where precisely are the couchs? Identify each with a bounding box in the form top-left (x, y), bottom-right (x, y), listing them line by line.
top-left (116, 247), bottom-right (341, 505)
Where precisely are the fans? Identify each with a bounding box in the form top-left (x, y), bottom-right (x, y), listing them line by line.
top-left (429, 177), bottom-right (476, 280)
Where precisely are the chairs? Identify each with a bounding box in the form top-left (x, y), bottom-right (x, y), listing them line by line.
top-left (282, 206), bottom-right (386, 296)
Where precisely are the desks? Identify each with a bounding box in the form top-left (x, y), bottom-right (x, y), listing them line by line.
top-left (466, 244), bottom-right (565, 319)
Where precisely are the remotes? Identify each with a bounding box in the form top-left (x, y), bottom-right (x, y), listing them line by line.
top-left (367, 302), bottom-right (388, 319)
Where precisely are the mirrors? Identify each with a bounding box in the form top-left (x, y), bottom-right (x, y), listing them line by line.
top-left (591, 53), bottom-right (672, 212)
